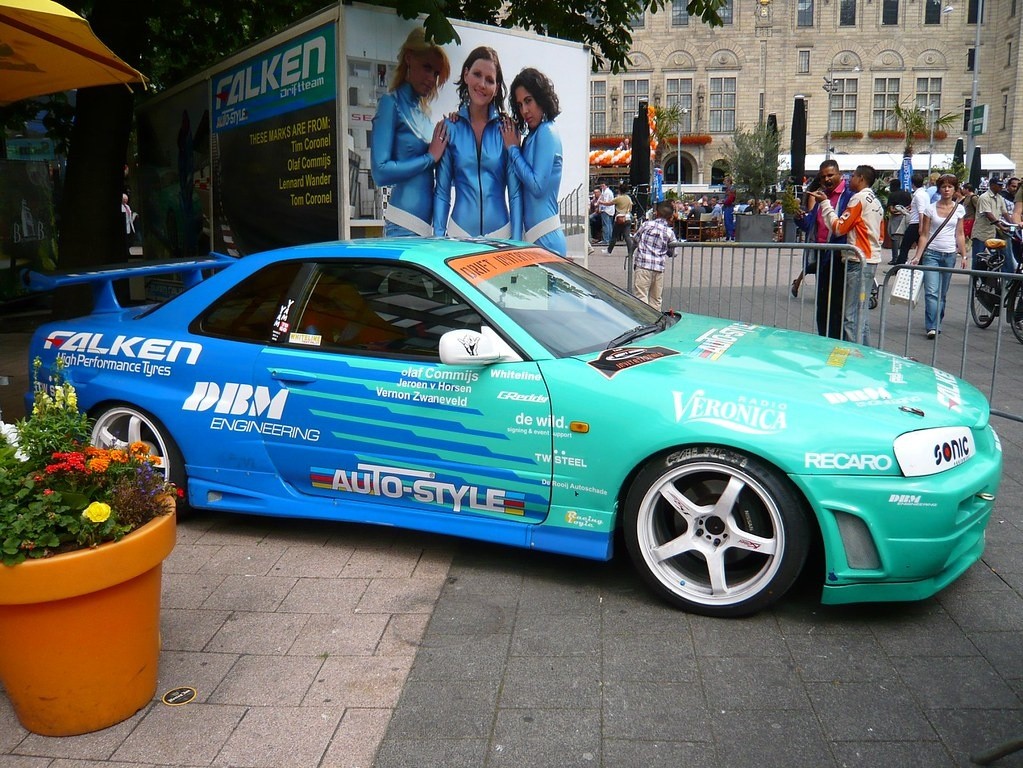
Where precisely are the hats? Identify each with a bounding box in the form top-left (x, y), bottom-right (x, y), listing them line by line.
top-left (990, 176), bottom-right (1007, 184)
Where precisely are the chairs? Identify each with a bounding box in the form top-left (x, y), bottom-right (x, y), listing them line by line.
top-left (685, 215), bottom-right (723, 242)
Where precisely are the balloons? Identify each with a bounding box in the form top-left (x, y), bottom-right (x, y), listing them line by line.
top-left (647, 106), bottom-right (659, 160)
top-left (589, 150), bottom-right (632, 164)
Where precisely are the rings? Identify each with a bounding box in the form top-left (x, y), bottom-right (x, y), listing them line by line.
top-left (440, 137), bottom-right (443, 140)
top-left (508, 126), bottom-right (511, 129)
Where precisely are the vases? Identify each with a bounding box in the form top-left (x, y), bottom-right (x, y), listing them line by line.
top-left (0, 487), bottom-right (177, 738)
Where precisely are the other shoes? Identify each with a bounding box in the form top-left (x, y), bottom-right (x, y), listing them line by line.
top-left (601, 248), bottom-right (612, 256)
top-left (598, 240), bottom-right (604, 244)
top-left (592, 238), bottom-right (599, 244)
top-left (888, 261), bottom-right (897, 265)
top-left (927, 330), bottom-right (936, 337)
top-left (588, 247), bottom-right (594, 255)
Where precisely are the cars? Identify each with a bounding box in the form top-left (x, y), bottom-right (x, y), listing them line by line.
top-left (21, 236), bottom-right (1006, 620)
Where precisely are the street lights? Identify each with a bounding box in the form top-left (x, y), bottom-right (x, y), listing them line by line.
top-left (672, 106), bottom-right (688, 198)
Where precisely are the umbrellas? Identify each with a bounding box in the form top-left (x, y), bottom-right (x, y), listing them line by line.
top-left (0, 0), bottom-right (150, 107)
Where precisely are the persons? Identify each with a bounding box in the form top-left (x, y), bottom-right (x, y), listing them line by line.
top-left (722, 178), bottom-right (805, 242)
top-left (886, 172), bottom-right (1023, 330)
top-left (646, 196), bottom-right (723, 242)
top-left (909, 174), bottom-right (968, 339)
top-left (433, 46), bottom-right (523, 241)
top-left (370, 27), bottom-right (460, 237)
top-left (793, 160), bottom-right (858, 341)
top-left (588, 182), bottom-right (633, 255)
top-left (629, 201), bottom-right (678, 312)
top-left (792, 173), bottom-right (833, 297)
top-left (806, 165), bottom-right (884, 347)
top-left (121, 193), bottom-right (136, 262)
top-left (499, 68), bottom-right (567, 258)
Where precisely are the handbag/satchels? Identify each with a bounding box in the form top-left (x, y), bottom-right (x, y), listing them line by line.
top-left (616, 216), bottom-right (626, 224)
top-left (911, 253), bottom-right (923, 266)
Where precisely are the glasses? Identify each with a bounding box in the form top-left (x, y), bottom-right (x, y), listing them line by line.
top-left (939, 174), bottom-right (956, 178)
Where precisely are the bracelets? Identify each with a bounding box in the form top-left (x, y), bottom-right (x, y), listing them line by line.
top-left (963, 256), bottom-right (967, 260)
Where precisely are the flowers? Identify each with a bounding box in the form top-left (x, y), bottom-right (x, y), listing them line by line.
top-left (0, 353), bottom-right (176, 565)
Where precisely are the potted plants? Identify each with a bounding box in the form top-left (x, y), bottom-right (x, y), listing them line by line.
top-left (876, 188), bottom-right (917, 249)
top-left (720, 119), bottom-right (788, 241)
top-left (781, 185), bottom-right (801, 242)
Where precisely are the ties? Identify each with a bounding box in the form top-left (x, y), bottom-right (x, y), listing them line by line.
top-left (124, 204), bottom-right (130, 215)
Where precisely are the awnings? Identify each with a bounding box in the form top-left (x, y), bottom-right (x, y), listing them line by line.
top-left (763, 154), bottom-right (1016, 170)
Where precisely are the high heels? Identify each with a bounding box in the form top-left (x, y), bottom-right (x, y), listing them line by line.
top-left (791, 279), bottom-right (799, 297)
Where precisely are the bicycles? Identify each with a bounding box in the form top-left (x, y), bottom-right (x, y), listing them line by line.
top-left (630, 183), bottom-right (655, 220)
top-left (969, 220), bottom-right (1023, 343)
